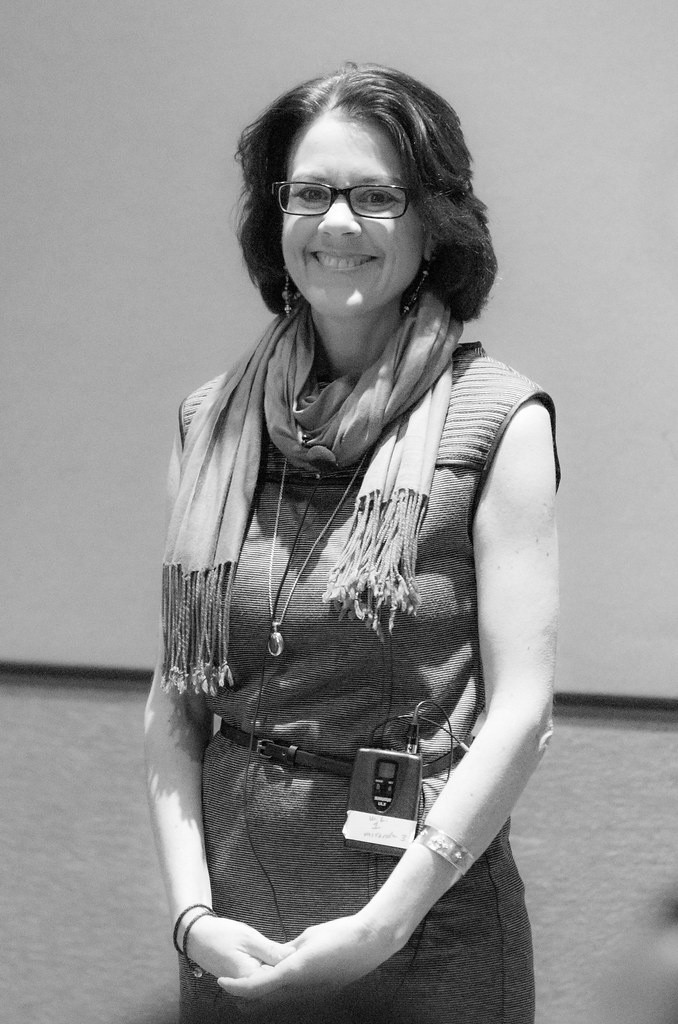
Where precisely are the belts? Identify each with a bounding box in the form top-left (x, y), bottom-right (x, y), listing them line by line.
top-left (219, 717), bottom-right (474, 779)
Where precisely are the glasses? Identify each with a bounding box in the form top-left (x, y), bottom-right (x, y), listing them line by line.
top-left (271, 181), bottom-right (422, 219)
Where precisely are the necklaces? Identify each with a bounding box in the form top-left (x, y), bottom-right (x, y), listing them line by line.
top-left (267, 449), bottom-right (369, 656)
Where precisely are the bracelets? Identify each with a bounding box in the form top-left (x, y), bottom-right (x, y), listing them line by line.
top-left (183, 913), bottom-right (216, 977)
top-left (418, 825), bottom-right (474, 876)
top-left (173, 905), bottom-right (214, 956)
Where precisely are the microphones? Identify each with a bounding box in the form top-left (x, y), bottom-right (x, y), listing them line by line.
top-left (310, 458), bottom-right (342, 474)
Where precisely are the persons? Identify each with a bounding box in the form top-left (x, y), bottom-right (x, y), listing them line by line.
top-left (144, 63), bottom-right (562, 1024)
